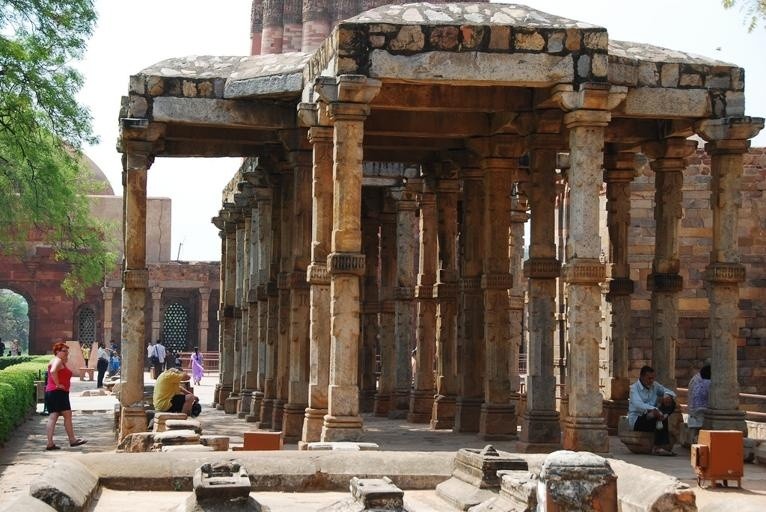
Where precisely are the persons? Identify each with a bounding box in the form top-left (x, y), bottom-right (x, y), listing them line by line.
top-left (628, 366), bottom-right (679, 457)
top-left (190, 347), bottom-right (204, 385)
top-left (687, 358), bottom-right (711, 428)
top-left (692, 365), bottom-right (711, 417)
top-left (80, 339), bottom-right (121, 389)
top-left (147, 340), bottom-right (182, 380)
top-left (45, 344), bottom-right (88, 449)
top-left (153, 368), bottom-right (199, 415)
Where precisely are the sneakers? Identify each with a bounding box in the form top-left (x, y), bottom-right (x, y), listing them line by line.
top-left (652, 446), bottom-right (677, 456)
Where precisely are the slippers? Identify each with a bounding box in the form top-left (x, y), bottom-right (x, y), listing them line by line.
top-left (46, 445), bottom-right (60, 450)
top-left (69, 439), bottom-right (88, 446)
